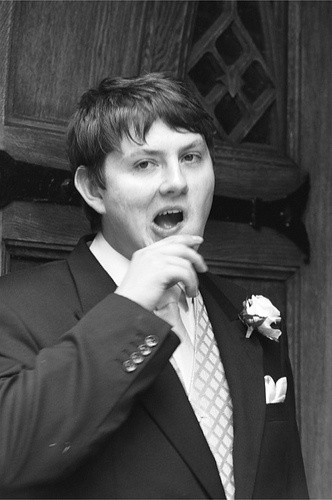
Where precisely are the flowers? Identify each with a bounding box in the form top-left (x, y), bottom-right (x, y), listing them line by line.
top-left (236, 293), bottom-right (282, 342)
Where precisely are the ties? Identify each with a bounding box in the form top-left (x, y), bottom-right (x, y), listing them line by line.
top-left (159, 300), bottom-right (193, 394)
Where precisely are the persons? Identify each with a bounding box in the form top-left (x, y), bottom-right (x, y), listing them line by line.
top-left (0, 70), bottom-right (310, 500)
